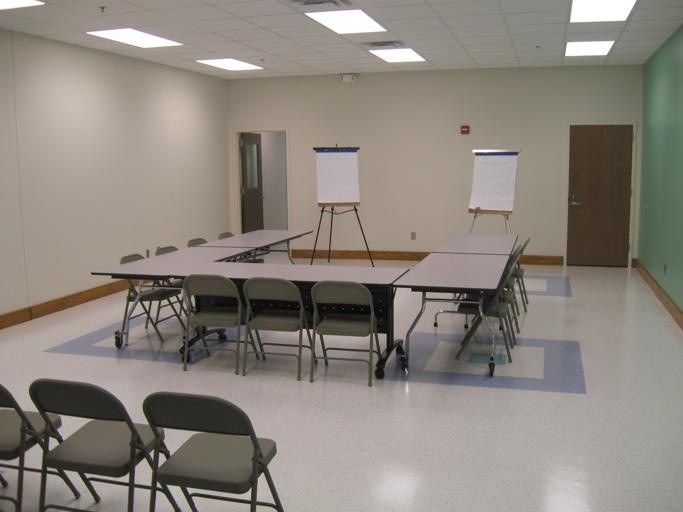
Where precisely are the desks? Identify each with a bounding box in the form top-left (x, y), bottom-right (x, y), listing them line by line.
top-left (174, 260), bottom-right (406, 380)
top-left (201, 229), bottom-right (314, 264)
top-left (432, 232), bottom-right (518, 330)
top-left (90, 245), bottom-right (256, 350)
top-left (392, 253), bottom-right (510, 379)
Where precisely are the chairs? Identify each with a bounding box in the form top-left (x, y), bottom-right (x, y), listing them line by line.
top-left (0, 384), bottom-right (80, 510)
top-left (181, 277), bottom-right (259, 374)
top-left (144, 246), bottom-right (202, 333)
top-left (28, 377), bottom-right (179, 511)
top-left (143, 391), bottom-right (283, 512)
top-left (240, 277), bottom-right (317, 381)
top-left (187, 236), bottom-right (208, 248)
top-left (218, 230), bottom-right (264, 263)
top-left (309, 283), bottom-right (383, 389)
top-left (474, 247), bottom-right (521, 317)
top-left (462, 247), bottom-right (520, 347)
top-left (455, 255), bottom-right (523, 364)
top-left (507, 237), bottom-right (534, 313)
top-left (121, 255), bottom-right (186, 347)
